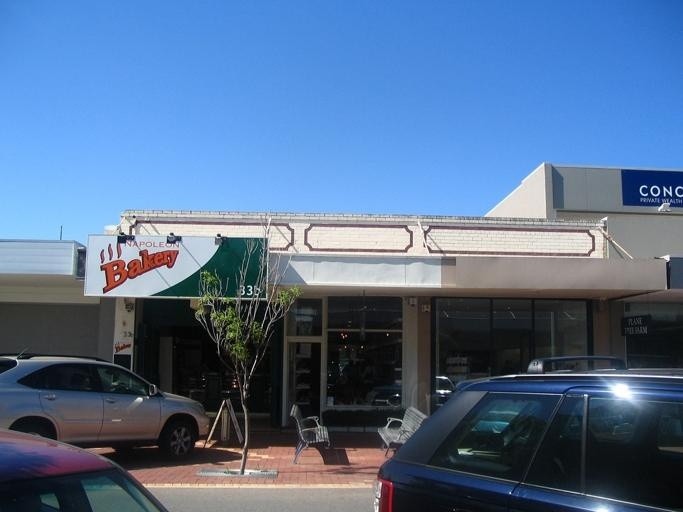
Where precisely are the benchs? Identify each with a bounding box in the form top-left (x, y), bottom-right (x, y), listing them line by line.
top-left (290, 405), bottom-right (331, 463)
top-left (378, 407), bottom-right (428, 456)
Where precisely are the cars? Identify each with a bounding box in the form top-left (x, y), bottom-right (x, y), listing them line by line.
top-left (0, 353), bottom-right (211, 511)
top-left (366, 368), bottom-right (402, 406)
top-left (373, 355), bottom-right (683, 512)
top-left (435, 376), bottom-right (455, 404)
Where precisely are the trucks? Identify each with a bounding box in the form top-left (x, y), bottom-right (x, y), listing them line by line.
top-left (172, 326), bottom-right (234, 398)
